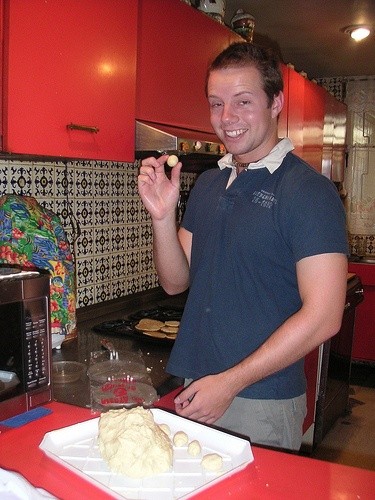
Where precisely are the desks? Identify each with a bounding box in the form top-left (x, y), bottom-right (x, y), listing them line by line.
top-left (0, 401), bottom-right (374, 500)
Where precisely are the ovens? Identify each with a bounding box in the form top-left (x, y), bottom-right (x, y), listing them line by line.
top-left (0, 263), bottom-right (53, 422)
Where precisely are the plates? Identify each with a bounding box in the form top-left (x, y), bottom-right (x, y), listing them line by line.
top-left (39, 408), bottom-right (254, 500)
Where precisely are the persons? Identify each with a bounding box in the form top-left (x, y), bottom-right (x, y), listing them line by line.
top-left (137, 44), bottom-right (347, 455)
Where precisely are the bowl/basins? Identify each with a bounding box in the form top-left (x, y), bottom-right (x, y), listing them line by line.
top-left (86, 350), bottom-right (158, 409)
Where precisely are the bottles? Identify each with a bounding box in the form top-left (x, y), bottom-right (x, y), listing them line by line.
top-left (53, 362), bottom-right (90, 407)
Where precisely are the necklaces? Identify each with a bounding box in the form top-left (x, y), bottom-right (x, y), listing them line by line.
top-left (233, 155), bottom-right (265, 167)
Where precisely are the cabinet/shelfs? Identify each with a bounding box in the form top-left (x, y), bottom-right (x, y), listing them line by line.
top-left (134, 264), bottom-right (375, 452)
top-left (0, 0), bottom-right (348, 182)
